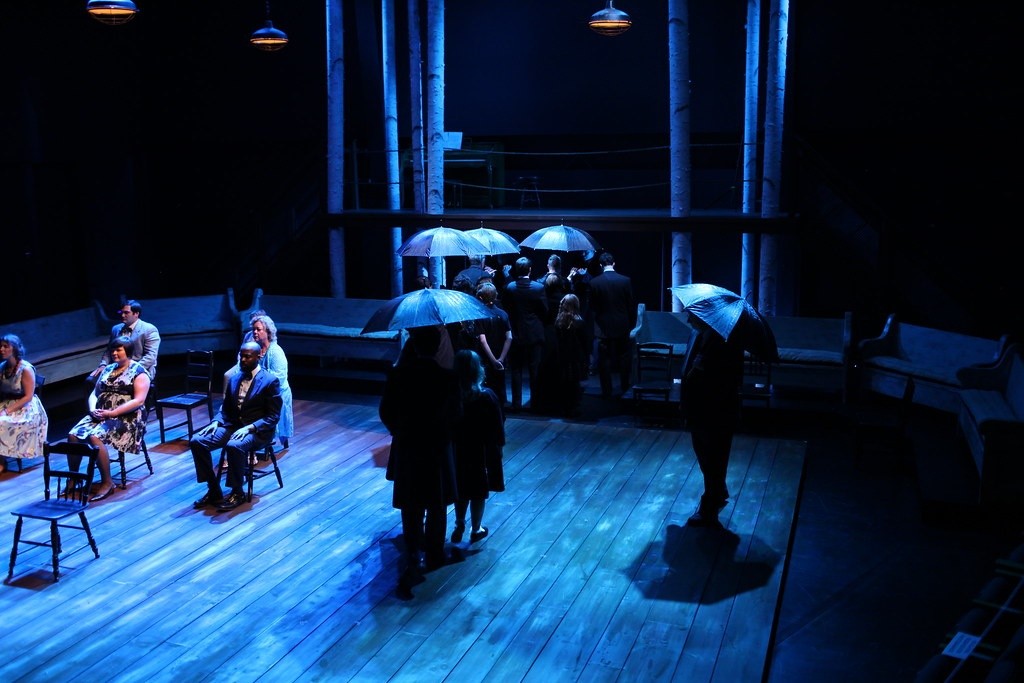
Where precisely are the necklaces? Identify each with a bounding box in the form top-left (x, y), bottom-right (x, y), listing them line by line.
top-left (5, 359), bottom-right (19, 379)
top-left (115, 367), bottom-right (116, 376)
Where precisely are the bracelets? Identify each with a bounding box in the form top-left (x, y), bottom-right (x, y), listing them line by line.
top-left (4, 409), bottom-right (8, 416)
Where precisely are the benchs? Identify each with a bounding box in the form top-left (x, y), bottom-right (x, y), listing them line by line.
top-left (118, 288), bottom-right (239, 356)
top-left (239, 288), bottom-right (408, 367)
top-left (859, 312), bottom-right (1010, 416)
top-left (629, 302), bottom-right (852, 405)
top-left (960, 344), bottom-right (1024, 483)
top-left (0, 300), bottom-right (121, 393)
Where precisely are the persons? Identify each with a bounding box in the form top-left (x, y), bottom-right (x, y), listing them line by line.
top-left (452, 254), bottom-right (637, 415)
top-left (190, 342), bottom-right (282, 511)
top-left (0, 334), bottom-right (48, 472)
top-left (379, 324), bottom-right (467, 600)
top-left (85, 299), bottom-right (161, 414)
top-left (224, 310), bottom-right (266, 395)
top-left (681, 312), bottom-right (742, 526)
top-left (450, 349), bottom-right (504, 543)
top-left (60, 336), bottom-right (152, 502)
top-left (223, 316), bottom-right (293, 468)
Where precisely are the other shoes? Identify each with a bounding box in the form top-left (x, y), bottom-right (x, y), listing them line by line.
top-left (522, 405), bottom-right (544, 417)
top-left (451, 524), bottom-right (465, 542)
top-left (222, 457), bottom-right (229, 468)
top-left (471, 525), bottom-right (488, 541)
top-left (406, 551), bottom-right (418, 567)
top-left (247, 451), bottom-right (259, 465)
top-left (89, 482), bottom-right (116, 501)
top-left (59, 478), bottom-right (83, 496)
top-left (426, 547), bottom-right (452, 569)
top-left (505, 405), bottom-right (520, 413)
top-left (688, 483), bottom-right (729, 526)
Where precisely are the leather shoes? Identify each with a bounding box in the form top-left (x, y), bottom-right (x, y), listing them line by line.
top-left (217, 491), bottom-right (246, 511)
top-left (194, 486), bottom-right (223, 506)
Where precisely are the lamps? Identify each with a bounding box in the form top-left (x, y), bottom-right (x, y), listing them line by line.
top-left (85, 0), bottom-right (140, 25)
top-left (250, 0), bottom-right (289, 45)
top-left (588, 0), bottom-right (633, 36)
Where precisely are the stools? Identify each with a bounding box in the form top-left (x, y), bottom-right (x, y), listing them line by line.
top-left (519, 177), bottom-right (544, 209)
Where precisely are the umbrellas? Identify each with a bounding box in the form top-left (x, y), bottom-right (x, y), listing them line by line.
top-left (360, 285), bottom-right (500, 336)
top-left (395, 226), bottom-right (492, 285)
top-left (518, 218), bottom-right (604, 256)
top-left (465, 220), bottom-right (521, 254)
top-left (670, 282), bottom-right (779, 361)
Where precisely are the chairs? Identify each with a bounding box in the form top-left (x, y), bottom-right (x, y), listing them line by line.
top-left (739, 345), bottom-right (773, 423)
top-left (3, 440), bottom-right (99, 588)
top-left (87, 385), bottom-right (156, 488)
top-left (156, 348), bottom-right (217, 443)
top-left (631, 342), bottom-right (673, 430)
top-left (215, 437), bottom-right (284, 500)
